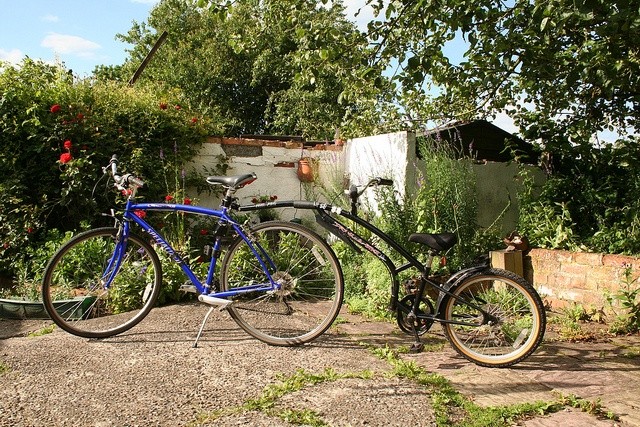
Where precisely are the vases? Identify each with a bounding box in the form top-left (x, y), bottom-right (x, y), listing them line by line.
top-left (298, 160), bottom-right (318, 183)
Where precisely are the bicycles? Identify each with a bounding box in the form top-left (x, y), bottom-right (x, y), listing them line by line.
top-left (40, 153), bottom-right (345, 348)
top-left (238, 176), bottom-right (547, 369)
